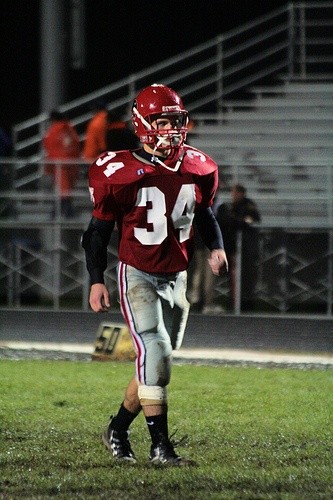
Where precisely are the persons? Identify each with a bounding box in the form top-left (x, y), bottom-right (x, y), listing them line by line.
top-left (44, 110), bottom-right (80, 218)
top-left (82, 101), bottom-right (127, 177)
top-left (218, 184), bottom-right (261, 224)
top-left (82, 82), bottom-right (229, 469)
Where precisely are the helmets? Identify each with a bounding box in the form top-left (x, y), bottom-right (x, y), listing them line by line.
top-left (131, 84), bottom-right (189, 148)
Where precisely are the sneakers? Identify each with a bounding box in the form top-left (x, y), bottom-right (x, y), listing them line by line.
top-left (101, 415), bottom-right (134, 461)
top-left (149, 440), bottom-right (193, 467)
